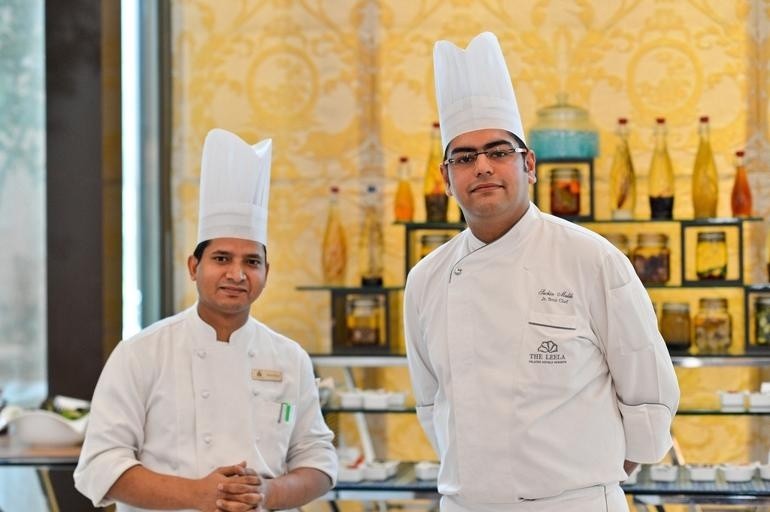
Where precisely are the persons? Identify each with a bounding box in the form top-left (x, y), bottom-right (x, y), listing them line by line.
top-left (73, 236), bottom-right (338, 512)
top-left (401, 129), bottom-right (682, 512)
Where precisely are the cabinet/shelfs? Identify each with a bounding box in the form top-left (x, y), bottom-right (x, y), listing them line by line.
top-left (298, 218), bottom-right (770, 512)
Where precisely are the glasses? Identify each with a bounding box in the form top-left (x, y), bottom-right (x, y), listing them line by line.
top-left (442, 149), bottom-right (527, 169)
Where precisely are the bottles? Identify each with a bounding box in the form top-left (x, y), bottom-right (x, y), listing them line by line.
top-left (345, 297), bottom-right (380, 346)
top-left (356, 185), bottom-right (384, 286)
top-left (730, 150), bottom-right (753, 218)
top-left (549, 117), bottom-right (638, 220)
top-left (598, 229), bottom-right (769, 351)
top-left (690, 115), bottom-right (719, 221)
top-left (320, 186), bottom-right (348, 289)
top-left (419, 121), bottom-right (449, 259)
top-left (645, 117), bottom-right (677, 221)
top-left (391, 156), bottom-right (415, 222)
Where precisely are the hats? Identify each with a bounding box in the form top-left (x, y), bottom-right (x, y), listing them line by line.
top-left (196, 129), bottom-right (272, 246)
top-left (433, 31), bottom-right (526, 155)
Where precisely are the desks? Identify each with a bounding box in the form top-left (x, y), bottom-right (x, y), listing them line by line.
top-left (0, 435), bottom-right (81, 512)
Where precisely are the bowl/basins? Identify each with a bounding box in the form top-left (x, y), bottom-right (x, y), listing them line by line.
top-left (339, 459), bottom-right (406, 484)
top-left (336, 390), bottom-right (411, 411)
top-left (413, 461), bottom-right (439, 481)
top-left (713, 389), bottom-right (748, 411)
top-left (624, 462), bottom-right (769, 485)
top-left (745, 392), bottom-right (769, 413)
top-left (2, 402), bottom-right (92, 448)
